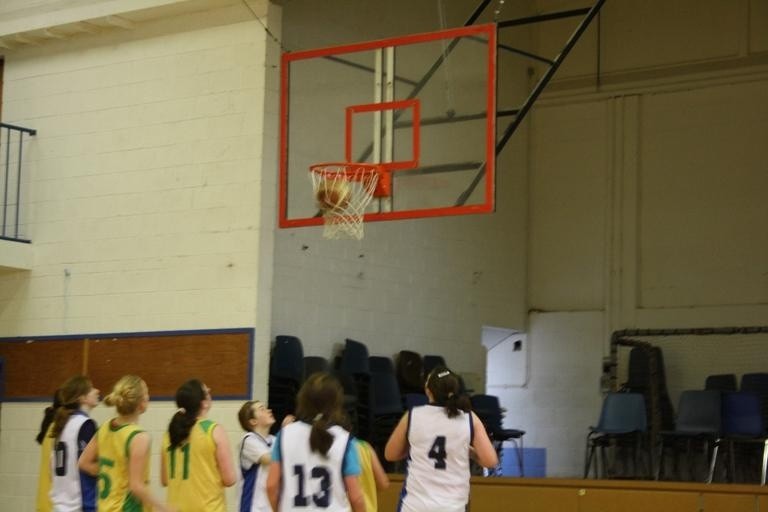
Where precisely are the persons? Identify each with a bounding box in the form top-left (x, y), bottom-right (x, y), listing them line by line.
top-left (77, 375), bottom-right (164, 512)
top-left (34, 387), bottom-right (61, 512)
top-left (159, 378), bottom-right (236, 512)
top-left (238, 400), bottom-right (296, 512)
top-left (265, 371), bottom-right (366, 512)
top-left (342, 395), bottom-right (390, 512)
top-left (384, 365), bottom-right (499, 512)
top-left (47, 376), bottom-right (101, 512)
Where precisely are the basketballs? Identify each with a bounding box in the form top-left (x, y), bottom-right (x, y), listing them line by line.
top-left (317, 174), bottom-right (351, 215)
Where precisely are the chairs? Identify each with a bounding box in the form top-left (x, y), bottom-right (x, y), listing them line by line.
top-left (578, 347), bottom-right (766, 484)
top-left (268, 336), bottom-right (523, 476)
